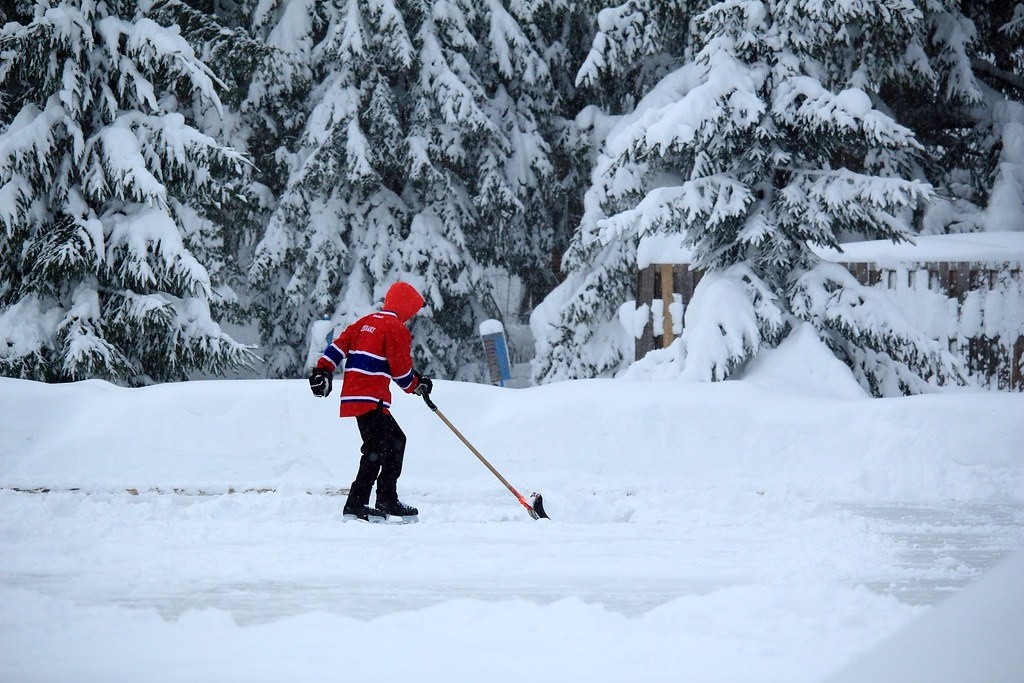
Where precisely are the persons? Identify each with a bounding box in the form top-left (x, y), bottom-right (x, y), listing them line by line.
top-left (310, 281), bottom-right (433, 522)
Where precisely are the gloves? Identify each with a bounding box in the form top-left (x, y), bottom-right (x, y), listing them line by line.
top-left (309, 366), bottom-right (333, 399)
top-left (412, 375), bottom-right (432, 396)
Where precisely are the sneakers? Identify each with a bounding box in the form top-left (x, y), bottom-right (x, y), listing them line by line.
top-left (374, 490), bottom-right (419, 525)
top-left (343, 486), bottom-right (387, 524)
